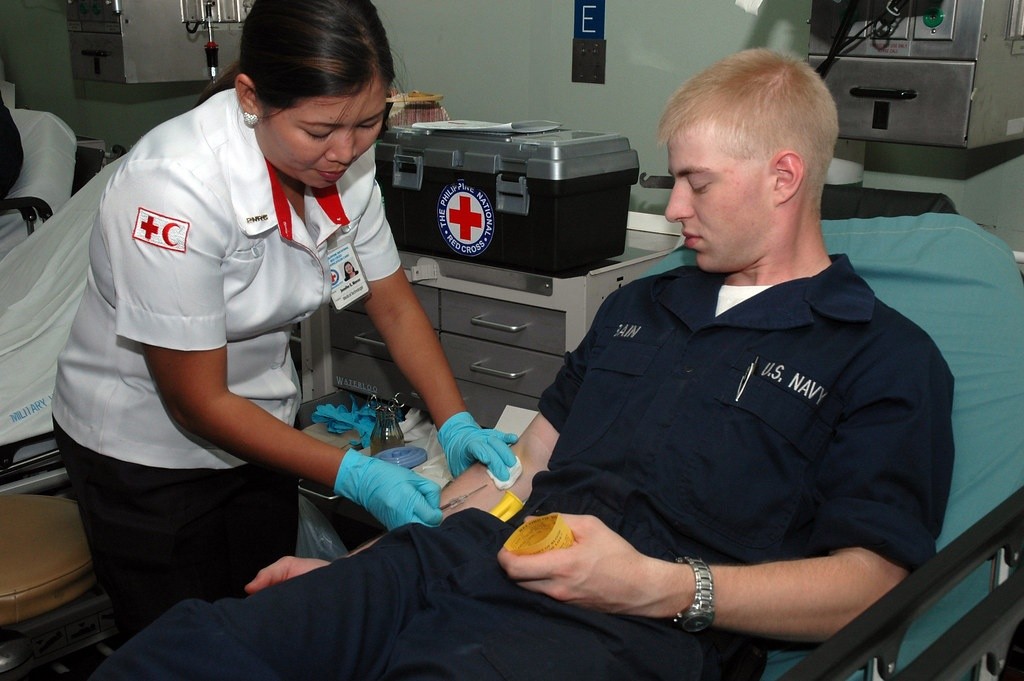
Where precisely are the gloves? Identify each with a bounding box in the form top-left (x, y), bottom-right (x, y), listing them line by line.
top-left (333, 448), bottom-right (443, 530)
top-left (312, 394), bottom-right (404, 450)
top-left (437, 412), bottom-right (519, 481)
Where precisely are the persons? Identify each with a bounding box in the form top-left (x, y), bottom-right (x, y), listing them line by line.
top-left (83, 49), bottom-right (956, 681)
top-left (51, 1), bottom-right (517, 640)
top-left (341, 261), bottom-right (358, 282)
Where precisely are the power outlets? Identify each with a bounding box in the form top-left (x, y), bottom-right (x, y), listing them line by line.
top-left (828, 0), bottom-right (957, 40)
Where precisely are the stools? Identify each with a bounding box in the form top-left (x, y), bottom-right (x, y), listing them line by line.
top-left (0, 491), bottom-right (99, 681)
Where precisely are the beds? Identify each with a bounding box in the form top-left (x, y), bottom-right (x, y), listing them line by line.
top-left (633, 213), bottom-right (1024, 681)
top-left (0, 103), bottom-right (128, 494)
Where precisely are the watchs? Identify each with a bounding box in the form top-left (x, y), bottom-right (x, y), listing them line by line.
top-left (669, 555), bottom-right (715, 633)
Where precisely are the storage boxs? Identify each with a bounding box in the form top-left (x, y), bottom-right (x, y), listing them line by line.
top-left (374, 119), bottom-right (639, 276)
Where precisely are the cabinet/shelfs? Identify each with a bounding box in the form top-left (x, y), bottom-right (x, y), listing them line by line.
top-left (311, 214), bottom-right (698, 438)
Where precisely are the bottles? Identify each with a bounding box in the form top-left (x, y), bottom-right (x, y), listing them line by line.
top-left (369, 407), bottom-right (405, 456)
